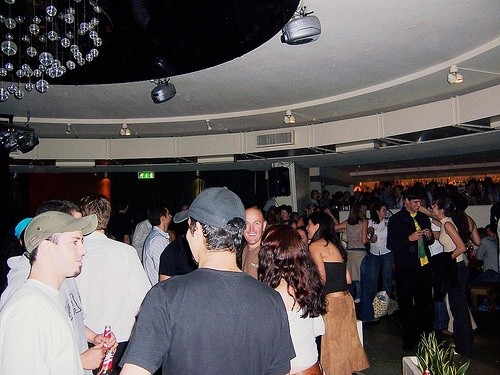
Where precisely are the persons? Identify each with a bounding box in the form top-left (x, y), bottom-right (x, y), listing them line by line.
top-left (0, 177), bottom-right (500, 375)
top-left (119, 189), bottom-right (297, 375)
top-left (0, 211), bottom-right (119, 375)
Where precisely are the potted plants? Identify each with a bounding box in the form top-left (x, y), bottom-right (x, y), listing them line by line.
top-left (402, 330), bottom-right (471, 375)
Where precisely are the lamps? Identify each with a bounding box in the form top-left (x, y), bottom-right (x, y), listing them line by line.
top-left (66, 122), bottom-right (79, 141)
top-left (120, 122), bottom-right (139, 138)
top-left (447, 64), bottom-right (464, 84)
top-left (204, 119), bottom-right (218, 132)
top-left (283, 108), bottom-right (296, 124)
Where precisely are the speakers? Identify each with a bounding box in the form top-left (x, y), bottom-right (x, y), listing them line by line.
top-left (151, 81), bottom-right (176, 104)
top-left (279, 15), bottom-right (321, 44)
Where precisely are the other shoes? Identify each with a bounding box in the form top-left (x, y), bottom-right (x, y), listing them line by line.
top-left (442, 328), bottom-right (452, 335)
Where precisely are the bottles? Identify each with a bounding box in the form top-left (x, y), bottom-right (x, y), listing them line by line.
top-left (93, 325), bottom-right (114, 375)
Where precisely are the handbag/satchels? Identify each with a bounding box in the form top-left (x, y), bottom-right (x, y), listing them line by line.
top-left (373, 291), bottom-right (390, 318)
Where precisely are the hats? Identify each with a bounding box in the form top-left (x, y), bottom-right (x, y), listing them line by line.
top-left (25, 211), bottom-right (98, 253)
top-left (173, 187), bottom-right (246, 231)
top-left (16, 218), bottom-right (32, 238)
